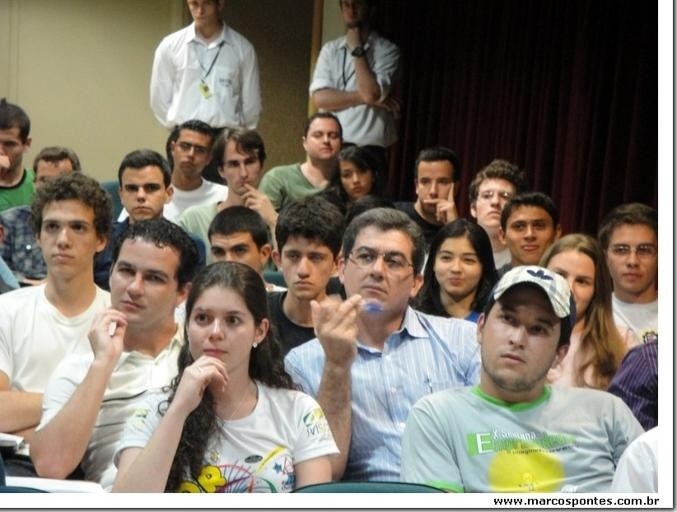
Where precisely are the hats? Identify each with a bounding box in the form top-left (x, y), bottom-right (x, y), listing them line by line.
top-left (487, 263), bottom-right (575, 330)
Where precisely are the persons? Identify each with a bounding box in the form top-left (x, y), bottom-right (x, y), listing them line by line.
top-left (0, 95), bottom-right (657, 494)
top-left (150, 1), bottom-right (262, 185)
top-left (309, 1), bottom-right (403, 195)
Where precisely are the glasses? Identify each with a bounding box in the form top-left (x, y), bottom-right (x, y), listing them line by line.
top-left (348, 247), bottom-right (416, 272)
top-left (606, 244), bottom-right (658, 258)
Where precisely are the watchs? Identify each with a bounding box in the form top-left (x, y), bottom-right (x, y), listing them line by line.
top-left (350, 45), bottom-right (368, 58)
